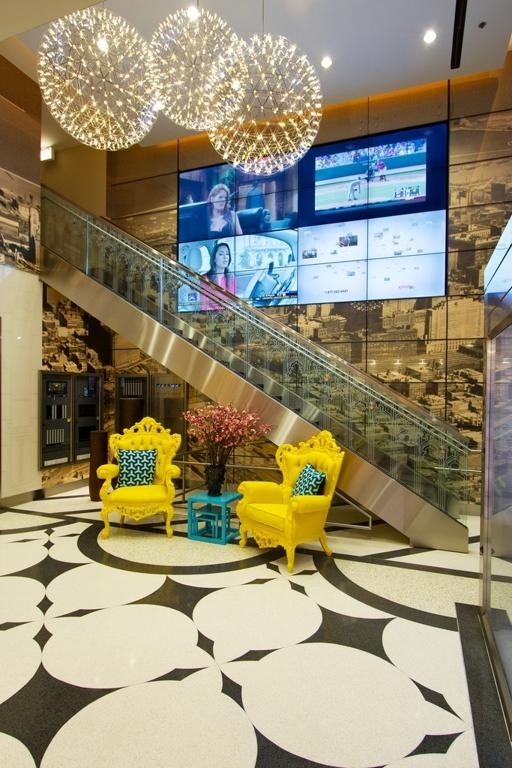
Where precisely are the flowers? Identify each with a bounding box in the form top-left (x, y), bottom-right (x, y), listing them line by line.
top-left (181, 402), bottom-right (275, 464)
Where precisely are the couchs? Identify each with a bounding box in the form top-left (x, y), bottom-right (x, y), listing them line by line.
top-left (97, 416), bottom-right (182, 540)
top-left (236, 430), bottom-right (344, 573)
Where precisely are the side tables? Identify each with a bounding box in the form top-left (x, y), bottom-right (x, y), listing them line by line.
top-left (188, 491), bottom-right (240, 545)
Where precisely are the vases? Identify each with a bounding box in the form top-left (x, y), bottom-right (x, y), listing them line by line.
top-left (203, 465), bottom-right (226, 496)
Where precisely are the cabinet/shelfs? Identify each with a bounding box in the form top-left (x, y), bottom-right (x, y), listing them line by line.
top-left (73, 372), bottom-right (102, 461)
top-left (39, 370), bottom-right (72, 467)
top-left (152, 373), bottom-right (189, 489)
top-left (115, 375), bottom-right (150, 433)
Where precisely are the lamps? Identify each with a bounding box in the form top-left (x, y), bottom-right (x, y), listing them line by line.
top-left (149, 0), bottom-right (250, 131)
top-left (36, 2), bottom-right (157, 150)
top-left (204, 2), bottom-right (323, 177)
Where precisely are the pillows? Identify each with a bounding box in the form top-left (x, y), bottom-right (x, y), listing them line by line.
top-left (116, 447), bottom-right (155, 487)
top-left (289, 465), bottom-right (326, 496)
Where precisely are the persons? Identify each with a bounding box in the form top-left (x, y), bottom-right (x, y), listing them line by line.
top-left (348, 176), bottom-right (362, 201)
top-left (200, 243), bottom-right (235, 311)
top-left (206, 184), bottom-right (243, 239)
top-left (246, 181), bottom-right (265, 210)
top-left (377, 160), bottom-right (388, 182)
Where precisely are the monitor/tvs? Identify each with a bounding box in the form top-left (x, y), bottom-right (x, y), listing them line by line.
top-left (177, 227), bottom-right (297, 312)
top-left (176, 149), bottom-right (299, 243)
top-left (298, 209), bottom-right (448, 305)
top-left (298, 119), bottom-right (450, 227)
